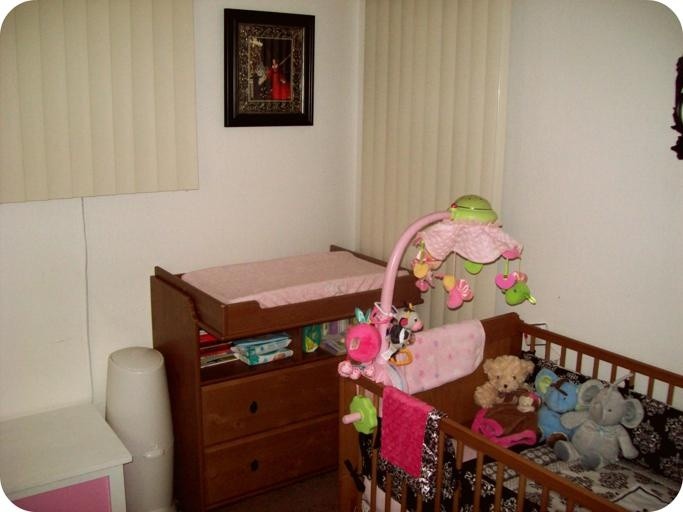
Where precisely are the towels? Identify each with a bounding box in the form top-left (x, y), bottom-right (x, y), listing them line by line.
top-left (381, 385), bottom-right (436, 477)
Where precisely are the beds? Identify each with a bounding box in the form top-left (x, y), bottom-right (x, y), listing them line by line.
top-left (336, 311), bottom-right (683, 512)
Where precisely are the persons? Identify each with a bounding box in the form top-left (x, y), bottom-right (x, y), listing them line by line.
top-left (268, 59), bottom-right (295, 103)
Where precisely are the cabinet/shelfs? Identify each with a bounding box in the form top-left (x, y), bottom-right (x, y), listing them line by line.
top-left (149, 244), bottom-right (423, 512)
top-left (1, 401), bottom-right (132, 511)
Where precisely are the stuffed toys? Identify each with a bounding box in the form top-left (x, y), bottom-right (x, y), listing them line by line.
top-left (532, 370), bottom-right (575, 443)
top-left (550, 371), bottom-right (640, 471)
top-left (410, 192), bottom-right (538, 313)
top-left (468, 356), bottom-right (543, 450)
top-left (337, 300), bottom-right (421, 381)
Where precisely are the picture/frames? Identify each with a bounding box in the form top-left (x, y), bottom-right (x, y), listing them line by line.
top-left (223, 9), bottom-right (315, 126)
